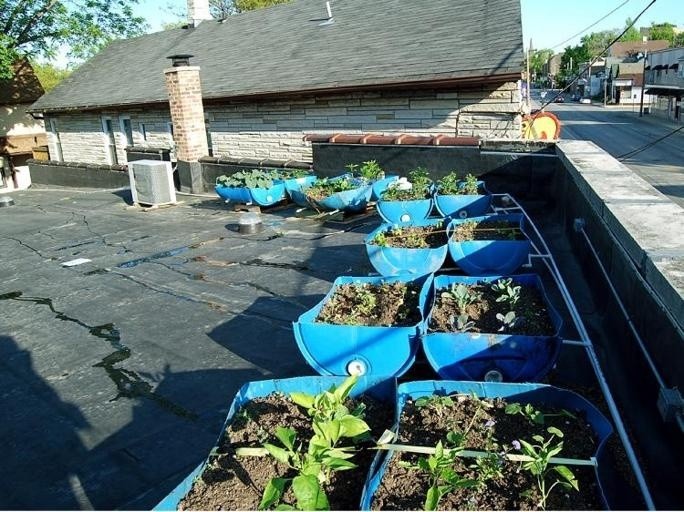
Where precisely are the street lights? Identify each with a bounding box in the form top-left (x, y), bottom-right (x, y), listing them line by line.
top-left (526, 47), bottom-right (538, 112)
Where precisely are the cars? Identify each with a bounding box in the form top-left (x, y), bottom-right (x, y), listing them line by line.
top-left (553, 94), bottom-right (565, 103)
top-left (570, 94), bottom-right (581, 102)
top-left (580, 98), bottom-right (590, 104)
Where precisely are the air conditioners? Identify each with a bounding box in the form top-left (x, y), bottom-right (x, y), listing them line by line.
top-left (127, 159), bottom-right (177, 207)
